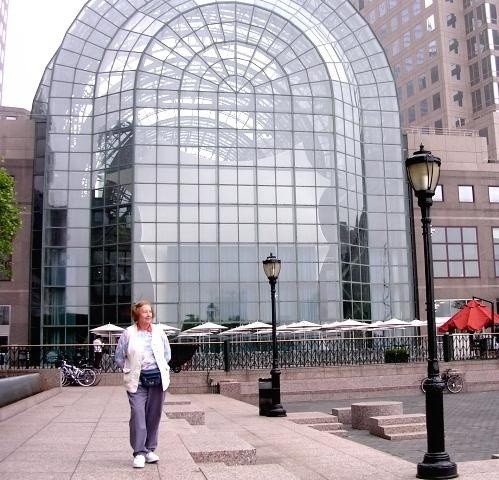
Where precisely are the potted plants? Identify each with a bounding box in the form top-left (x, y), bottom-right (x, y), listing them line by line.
top-left (382, 345), bottom-right (410, 364)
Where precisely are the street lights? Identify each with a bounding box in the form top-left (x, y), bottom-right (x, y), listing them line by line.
top-left (262, 251), bottom-right (290, 419)
top-left (402, 141), bottom-right (462, 480)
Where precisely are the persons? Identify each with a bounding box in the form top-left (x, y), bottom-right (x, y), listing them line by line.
top-left (93, 336), bottom-right (105, 369)
top-left (113, 301), bottom-right (172, 468)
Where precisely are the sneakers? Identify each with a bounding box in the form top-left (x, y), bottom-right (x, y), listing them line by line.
top-left (132, 454), bottom-right (145, 468)
top-left (145, 450), bottom-right (160, 463)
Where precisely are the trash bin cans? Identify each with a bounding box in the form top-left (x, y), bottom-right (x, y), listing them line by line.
top-left (258, 378), bottom-right (272, 415)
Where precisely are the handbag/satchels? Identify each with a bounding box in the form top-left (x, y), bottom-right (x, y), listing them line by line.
top-left (138, 369), bottom-right (162, 388)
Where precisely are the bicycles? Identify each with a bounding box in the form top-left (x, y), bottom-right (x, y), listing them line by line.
top-left (419, 365), bottom-right (464, 394)
top-left (53, 356), bottom-right (103, 388)
